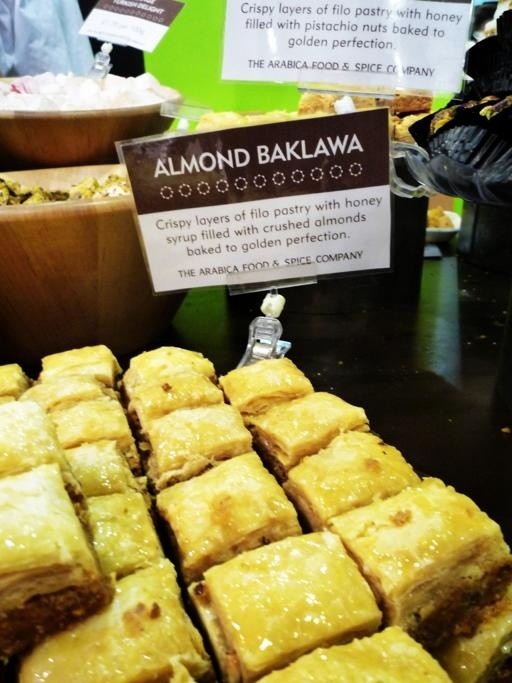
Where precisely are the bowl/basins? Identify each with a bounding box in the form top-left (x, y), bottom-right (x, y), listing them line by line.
top-left (0, 76), bottom-right (183, 171)
top-left (0, 166), bottom-right (189, 365)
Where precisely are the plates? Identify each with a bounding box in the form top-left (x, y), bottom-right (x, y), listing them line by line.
top-left (425, 208), bottom-right (462, 248)
